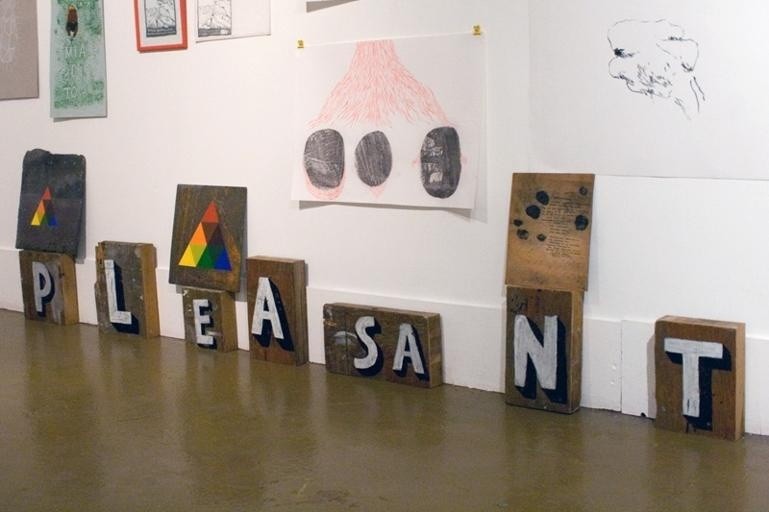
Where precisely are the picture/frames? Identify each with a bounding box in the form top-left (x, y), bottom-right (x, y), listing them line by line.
top-left (133, 1), bottom-right (188, 53)
